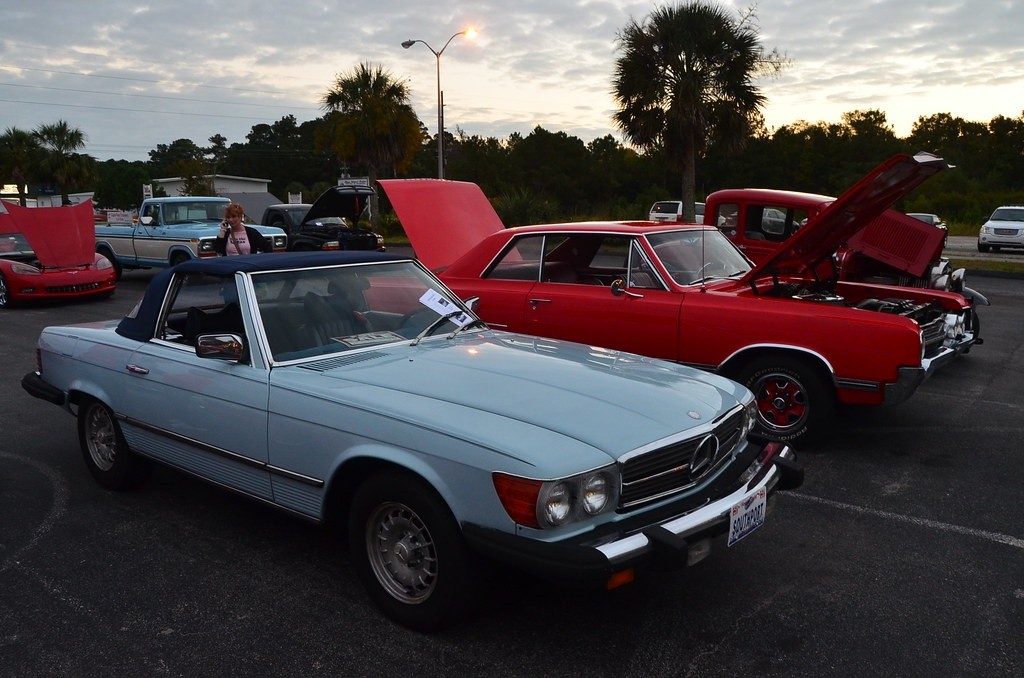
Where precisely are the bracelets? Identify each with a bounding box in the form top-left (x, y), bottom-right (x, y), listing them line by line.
top-left (220, 229), bottom-right (227, 232)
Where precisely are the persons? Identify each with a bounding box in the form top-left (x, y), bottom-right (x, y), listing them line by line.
top-left (215, 203), bottom-right (273, 307)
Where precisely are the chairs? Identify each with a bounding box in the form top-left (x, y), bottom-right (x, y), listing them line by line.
top-left (619, 273), bottom-right (654, 287)
top-left (267, 293), bottom-right (367, 356)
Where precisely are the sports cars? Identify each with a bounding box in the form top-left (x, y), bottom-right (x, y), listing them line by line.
top-left (0, 198), bottom-right (119, 308)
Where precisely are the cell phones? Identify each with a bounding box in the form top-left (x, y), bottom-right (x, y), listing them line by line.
top-left (225, 220), bottom-right (228, 227)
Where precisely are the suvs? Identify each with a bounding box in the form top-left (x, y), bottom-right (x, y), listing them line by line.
top-left (978, 206), bottom-right (1024, 253)
top-left (649, 200), bottom-right (727, 226)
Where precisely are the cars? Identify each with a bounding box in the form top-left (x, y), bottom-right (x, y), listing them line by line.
top-left (215, 185), bottom-right (387, 252)
top-left (725, 208), bottom-right (800, 236)
top-left (905, 213), bottom-right (949, 248)
top-left (372, 150), bottom-right (976, 449)
top-left (640, 189), bottom-right (992, 340)
top-left (22, 250), bottom-right (806, 634)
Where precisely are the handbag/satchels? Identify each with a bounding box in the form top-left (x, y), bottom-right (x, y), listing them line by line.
top-left (256, 281), bottom-right (270, 300)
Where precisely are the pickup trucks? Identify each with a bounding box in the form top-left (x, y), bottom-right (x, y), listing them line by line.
top-left (95, 197), bottom-right (288, 282)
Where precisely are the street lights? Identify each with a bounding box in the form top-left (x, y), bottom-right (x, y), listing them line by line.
top-left (401, 26), bottom-right (479, 180)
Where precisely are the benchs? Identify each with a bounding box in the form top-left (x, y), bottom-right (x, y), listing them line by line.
top-left (165, 302), bottom-right (283, 335)
